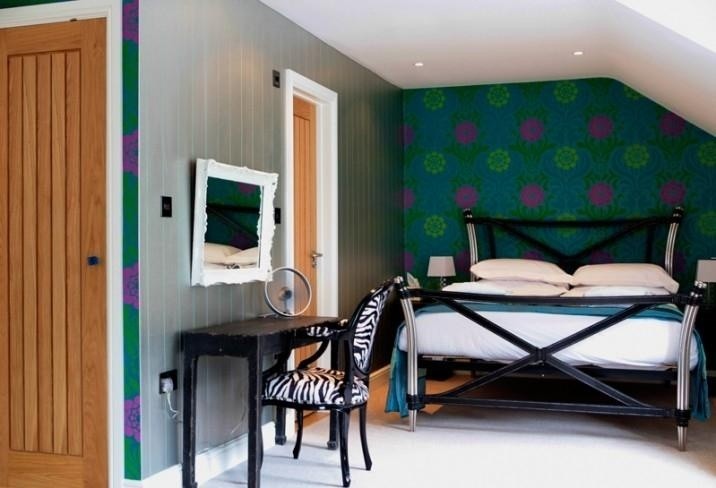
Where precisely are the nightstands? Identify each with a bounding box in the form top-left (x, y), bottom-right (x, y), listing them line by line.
top-left (679, 301), bottom-right (716, 397)
top-left (401, 293), bottom-right (437, 328)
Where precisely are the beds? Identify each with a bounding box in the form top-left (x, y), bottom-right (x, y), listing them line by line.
top-left (396, 206), bottom-right (708, 450)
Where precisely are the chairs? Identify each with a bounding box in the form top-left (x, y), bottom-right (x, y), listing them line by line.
top-left (259, 274), bottom-right (395, 487)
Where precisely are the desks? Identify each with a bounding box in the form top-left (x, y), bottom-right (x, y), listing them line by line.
top-left (181, 313), bottom-right (350, 488)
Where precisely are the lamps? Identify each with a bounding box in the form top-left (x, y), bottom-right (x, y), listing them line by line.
top-left (427, 256), bottom-right (456, 294)
top-left (694, 259), bottom-right (716, 309)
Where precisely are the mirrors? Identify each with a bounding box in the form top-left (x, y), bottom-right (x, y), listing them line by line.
top-left (190, 158), bottom-right (279, 288)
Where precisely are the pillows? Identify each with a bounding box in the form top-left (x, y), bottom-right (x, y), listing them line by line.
top-left (560, 287), bottom-right (669, 309)
top-left (570, 263), bottom-right (680, 295)
top-left (471, 259), bottom-right (574, 288)
top-left (204, 263), bottom-right (233, 271)
top-left (203, 242), bottom-right (241, 266)
top-left (440, 280), bottom-right (569, 304)
top-left (220, 247), bottom-right (259, 269)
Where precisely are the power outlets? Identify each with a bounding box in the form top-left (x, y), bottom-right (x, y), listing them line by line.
top-left (159, 369), bottom-right (177, 395)
